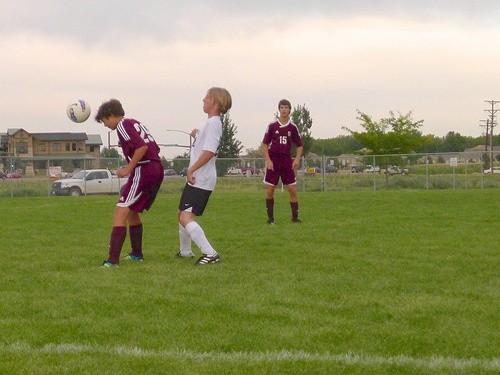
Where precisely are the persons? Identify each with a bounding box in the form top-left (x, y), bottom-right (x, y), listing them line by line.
top-left (261, 99), bottom-right (303, 224)
top-left (93, 99), bottom-right (164, 268)
top-left (174, 86), bottom-right (232, 264)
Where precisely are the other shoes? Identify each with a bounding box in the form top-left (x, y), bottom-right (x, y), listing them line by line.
top-left (291, 219), bottom-right (302, 223)
top-left (267, 220), bottom-right (274, 225)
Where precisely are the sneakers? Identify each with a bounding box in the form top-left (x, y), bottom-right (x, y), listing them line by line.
top-left (174, 251), bottom-right (196, 259)
top-left (96, 260), bottom-right (118, 268)
top-left (195, 252), bottom-right (220, 265)
top-left (121, 252), bottom-right (144, 262)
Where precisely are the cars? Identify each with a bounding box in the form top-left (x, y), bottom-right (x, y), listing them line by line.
top-left (242, 166), bottom-right (260, 175)
top-left (165, 169), bottom-right (175, 175)
top-left (315, 166), bottom-right (338, 174)
top-left (178, 170), bottom-right (187, 176)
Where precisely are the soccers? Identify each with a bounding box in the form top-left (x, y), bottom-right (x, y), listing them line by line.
top-left (65, 96), bottom-right (93, 124)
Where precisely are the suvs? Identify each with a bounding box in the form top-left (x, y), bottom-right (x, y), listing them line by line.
top-left (484, 167), bottom-right (500, 174)
top-left (366, 165), bottom-right (380, 173)
top-left (351, 165), bottom-right (363, 173)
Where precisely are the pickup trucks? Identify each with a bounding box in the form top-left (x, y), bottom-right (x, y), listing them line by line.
top-left (384, 166), bottom-right (409, 175)
top-left (227, 167), bottom-right (242, 175)
top-left (52, 168), bottom-right (130, 197)
